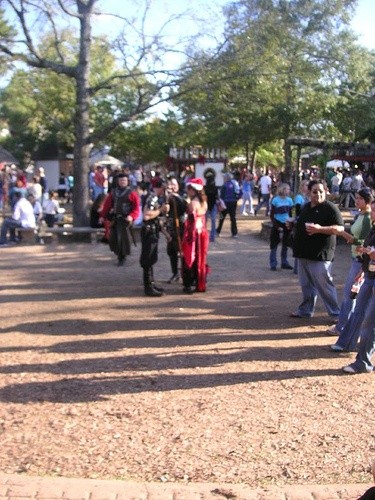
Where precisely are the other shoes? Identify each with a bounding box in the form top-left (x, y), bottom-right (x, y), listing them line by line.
top-left (167, 273), bottom-right (179, 283)
top-left (266, 213), bottom-right (270, 216)
top-left (282, 265), bottom-right (292, 269)
top-left (290, 314), bottom-right (302, 318)
top-left (215, 230), bottom-right (219, 237)
top-left (40, 238), bottom-right (45, 244)
top-left (255, 210), bottom-right (257, 215)
top-left (270, 266), bottom-right (276, 271)
top-left (249, 211), bottom-right (255, 214)
top-left (331, 344), bottom-right (344, 352)
top-left (327, 328), bottom-right (341, 336)
top-left (232, 234), bottom-right (239, 238)
top-left (8, 240), bottom-right (17, 245)
top-left (0, 242), bottom-right (8, 246)
top-left (117, 257), bottom-right (127, 266)
top-left (240, 212), bottom-right (248, 217)
top-left (343, 366), bottom-right (373, 373)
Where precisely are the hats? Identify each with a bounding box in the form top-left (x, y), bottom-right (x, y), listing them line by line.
top-left (232, 170), bottom-right (241, 175)
top-left (186, 178), bottom-right (204, 191)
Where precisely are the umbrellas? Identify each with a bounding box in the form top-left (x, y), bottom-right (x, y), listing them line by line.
top-left (88, 152), bottom-right (125, 165)
top-left (0, 147), bottom-right (20, 165)
top-left (326, 160), bottom-right (350, 168)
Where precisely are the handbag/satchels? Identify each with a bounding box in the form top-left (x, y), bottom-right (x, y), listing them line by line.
top-left (216, 198), bottom-right (227, 213)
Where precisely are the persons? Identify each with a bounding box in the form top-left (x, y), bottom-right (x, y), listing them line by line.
top-left (0, 164), bottom-right (375, 375)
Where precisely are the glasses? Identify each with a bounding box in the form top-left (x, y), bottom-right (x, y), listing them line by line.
top-left (313, 191), bottom-right (323, 194)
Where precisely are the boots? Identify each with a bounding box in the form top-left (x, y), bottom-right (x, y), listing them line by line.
top-left (143, 268), bottom-right (164, 296)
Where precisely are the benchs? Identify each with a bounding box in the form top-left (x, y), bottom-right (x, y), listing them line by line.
top-left (54, 221), bottom-right (74, 227)
top-left (16, 228), bottom-right (39, 245)
top-left (45, 226), bottom-right (106, 251)
top-left (259, 222), bottom-right (284, 241)
top-left (343, 219), bottom-right (355, 225)
top-left (132, 225), bottom-right (142, 243)
